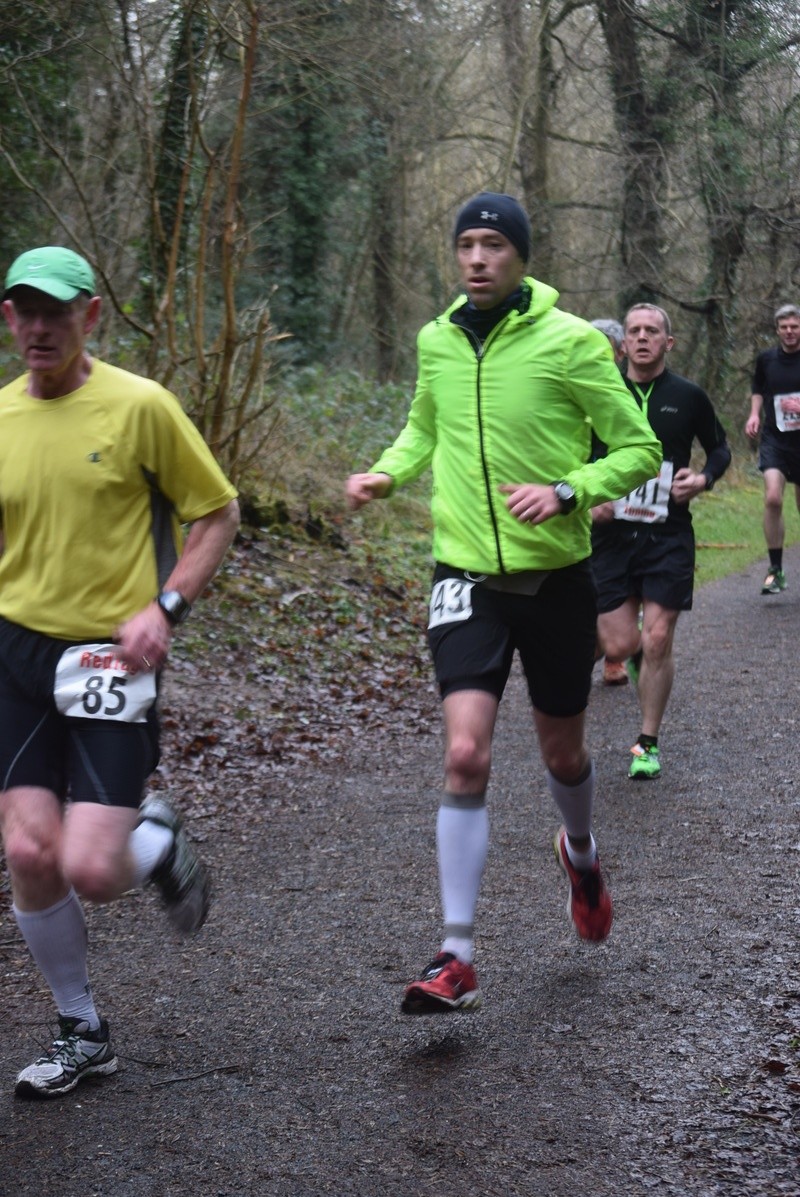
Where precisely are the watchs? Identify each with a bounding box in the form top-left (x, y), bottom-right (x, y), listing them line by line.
top-left (549, 481), bottom-right (576, 516)
top-left (156, 589), bottom-right (191, 626)
top-left (701, 471), bottom-right (715, 491)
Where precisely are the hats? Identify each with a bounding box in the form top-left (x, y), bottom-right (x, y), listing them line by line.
top-left (0, 247), bottom-right (97, 302)
top-left (454, 191), bottom-right (530, 264)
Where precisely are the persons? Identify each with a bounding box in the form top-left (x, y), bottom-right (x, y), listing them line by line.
top-left (590, 303), bottom-right (733, 777)
top-left (343, 192), bottom-right (666, 1015)
top-left (0, 246), bottom-right (242, 1095)
top-left (745, 306), bottom-right (800, 595)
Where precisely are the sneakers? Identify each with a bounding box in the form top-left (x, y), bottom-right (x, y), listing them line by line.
top-left (628, 622), bottom-right (645, 685)
top-left (138, 798), bottom-right (209, 933)
top-left (14, 1015), bottom-right (118, 1098)
top-left (604, 655), bottom-right (626, 685)
top-left (555, 825), bottom-right (614, 943)
top-left (399, 952), bottom-right (480, 1014)
top-left (761, 568), bottom-right (789, 595)
top-left (628, 741), bottom-right (662, 781)
